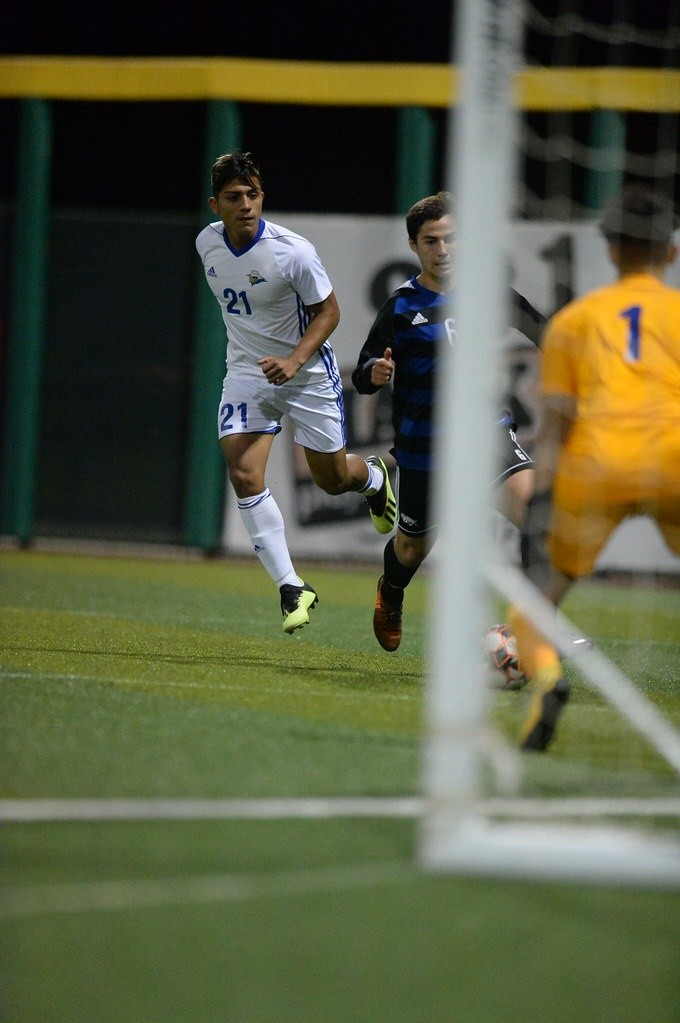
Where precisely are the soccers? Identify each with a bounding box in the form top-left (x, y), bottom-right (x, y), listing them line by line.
top-left (481, 624), bottom-right (533, 690)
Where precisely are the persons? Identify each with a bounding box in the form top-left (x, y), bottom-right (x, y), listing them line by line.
top-left (514, 184), bottom-right (680, 754)
top-left (351, 192), bottom-right (548, 652)
top-left (196, 152), bottom-right (397, 635)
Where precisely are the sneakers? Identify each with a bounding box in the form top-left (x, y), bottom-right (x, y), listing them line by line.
top-left (522, 667), bottom-right (569, 750)
top-left (365, 455), bottom-right (397, 534)
top-left (279, 580), bottom-right (318, 634)
top-left (373, 573), bottom-right (405, 652)
